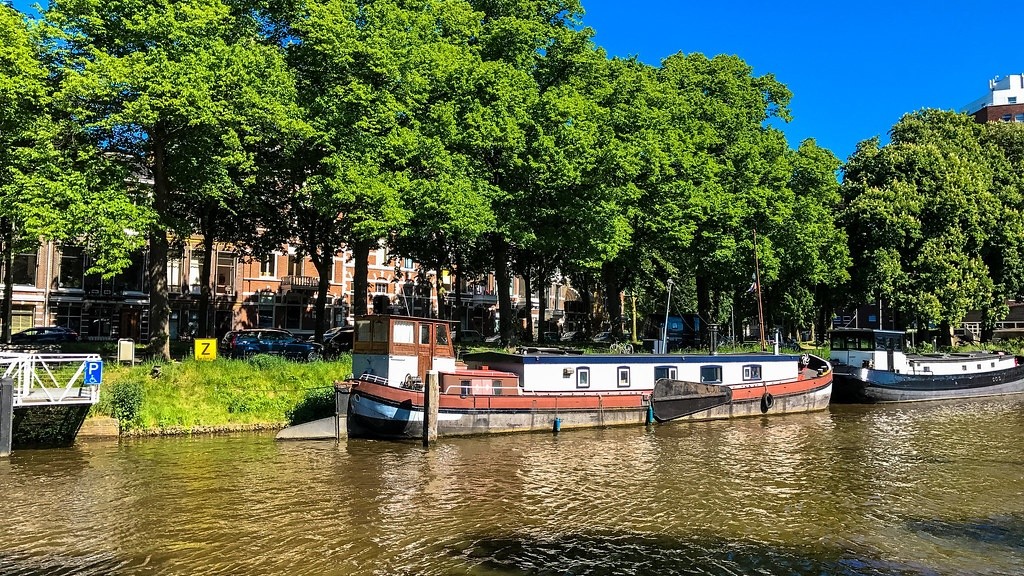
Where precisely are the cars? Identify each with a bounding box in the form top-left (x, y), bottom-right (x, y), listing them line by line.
top-left (560, 331), bottom-right (586, 343)
top-left (594, 331), bottom-right (615, 343)
top-left (308, 324), bottom-right (354, 360)
top-left (11, 326), bottom-right (77, 347)
top-left (544, 331), bottom-right (558, 343)
top-left (485, 331), bottom-right (502, 345)
top-left (442, 329), bottom-right (484, 345)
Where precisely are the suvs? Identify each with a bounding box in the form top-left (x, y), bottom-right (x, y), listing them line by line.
top-left (220, 327), bottom-right (325, 364)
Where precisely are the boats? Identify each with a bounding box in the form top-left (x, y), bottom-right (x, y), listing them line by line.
top-left (822, 328), bottom-right (1024, 405)
top-left (340, 312), bottom-right (835, 442)
